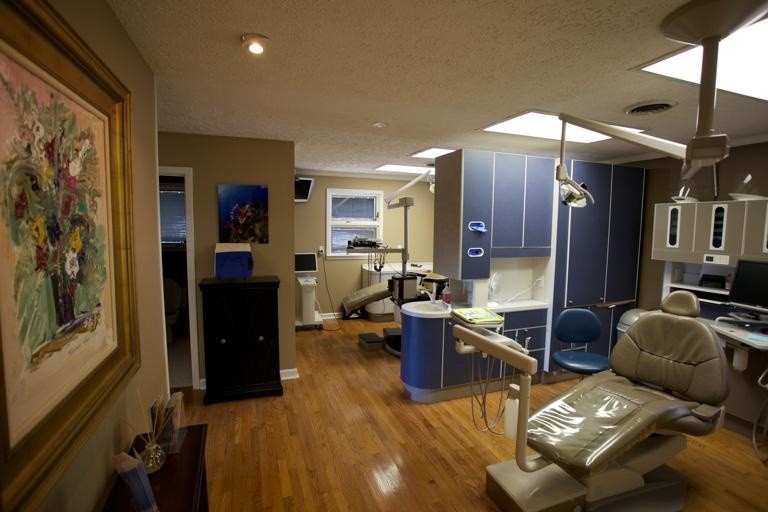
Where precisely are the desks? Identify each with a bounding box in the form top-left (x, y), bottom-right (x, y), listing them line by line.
top-left (696, 299), bottom-right (768, 351)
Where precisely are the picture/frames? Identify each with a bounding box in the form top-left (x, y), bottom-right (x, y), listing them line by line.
top-left (0, 0), bottom-right (141, 512)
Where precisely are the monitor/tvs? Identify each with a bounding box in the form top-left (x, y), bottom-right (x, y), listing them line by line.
top-left (294, 177), bottom-right (314, 203)
top-left (295, 253), bottom-right (318, 274)
top-left (727, 259), bottom-right (768, 318)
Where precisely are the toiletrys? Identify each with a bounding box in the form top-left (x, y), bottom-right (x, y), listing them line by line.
top-left (441, 282), bottom-right (449, 302)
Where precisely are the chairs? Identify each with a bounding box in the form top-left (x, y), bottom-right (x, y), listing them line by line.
top-left (528, 290), bottom-right (732, 474)
top-left (342, 269), bottom-right (433, 318)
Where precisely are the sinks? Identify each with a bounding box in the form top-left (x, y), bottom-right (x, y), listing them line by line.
top-left (401, 299), bottom-right (451, 317)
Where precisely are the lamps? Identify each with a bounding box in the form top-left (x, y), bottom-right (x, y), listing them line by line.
top-left (240, 32), bottom-right (272, 55)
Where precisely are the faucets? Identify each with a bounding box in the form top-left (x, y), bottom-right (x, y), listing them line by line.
top-left (421, 282), bottom-right (437, 303)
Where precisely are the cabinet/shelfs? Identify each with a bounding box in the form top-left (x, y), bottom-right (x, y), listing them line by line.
top-left (428, 148), bottom-right (492, 280)
top-left (543, 157), bottom-right (649, 383)
top-left (650, 199), bottom-right (768, 267)
top-left (102, 422), bottom-right (209, 512)
top-left (452, 301), bottom-right (544, 387)
top-left (489, 152), bottom-right (554, 257)
top-left (199, 275), bottom-right (283, 406)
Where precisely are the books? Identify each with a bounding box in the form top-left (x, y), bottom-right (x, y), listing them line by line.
top-left (148, 390), bottom-right (185, 454)
top-left (111, 444), bottom-right (160, 510)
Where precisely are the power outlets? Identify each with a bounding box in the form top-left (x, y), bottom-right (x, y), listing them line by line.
top-left (319, 246), bottom-right (324, 255)
